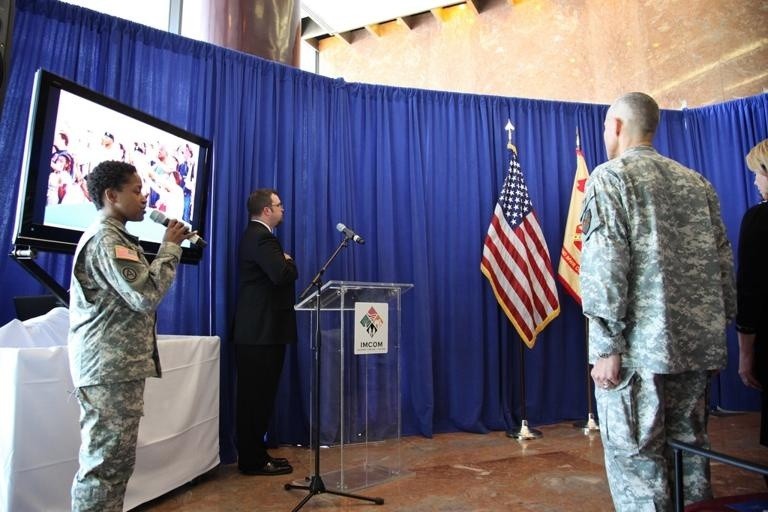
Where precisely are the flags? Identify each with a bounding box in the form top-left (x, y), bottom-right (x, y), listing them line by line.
top-left (550, 148), bottom-right (590, 304)
top-left (479, 140), bottom-right (561, 350)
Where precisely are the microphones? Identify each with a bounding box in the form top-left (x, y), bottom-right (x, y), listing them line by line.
top-left (336, 223), bottom-right (365, 246)
top-left (150, 210), bottom-right (208, 249)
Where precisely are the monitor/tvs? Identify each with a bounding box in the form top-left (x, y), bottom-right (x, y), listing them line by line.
top-left (12, 68), bottom-right (212, 265)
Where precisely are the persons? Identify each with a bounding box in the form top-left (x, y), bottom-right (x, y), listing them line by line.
top-left (67, 159), bottom-right (196, 512)
top-left (734, 136), bottom-right (768, 448)
top-left (45, 127), bottom-right (197, 225)
top-left (576, 92), bottom-right (737, 512)
top-left (232, 189), bottom-right (298, 477)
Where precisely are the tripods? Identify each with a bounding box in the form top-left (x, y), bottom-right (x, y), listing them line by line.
top-left (284, 244), bottom-right (385, 512)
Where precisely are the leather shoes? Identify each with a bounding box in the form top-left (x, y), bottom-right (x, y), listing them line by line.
top-left (238, 457), bottom-right (292, 476)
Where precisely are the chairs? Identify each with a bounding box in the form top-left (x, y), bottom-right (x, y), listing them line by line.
top-left (667, 437), bottom-right (768, 511)
